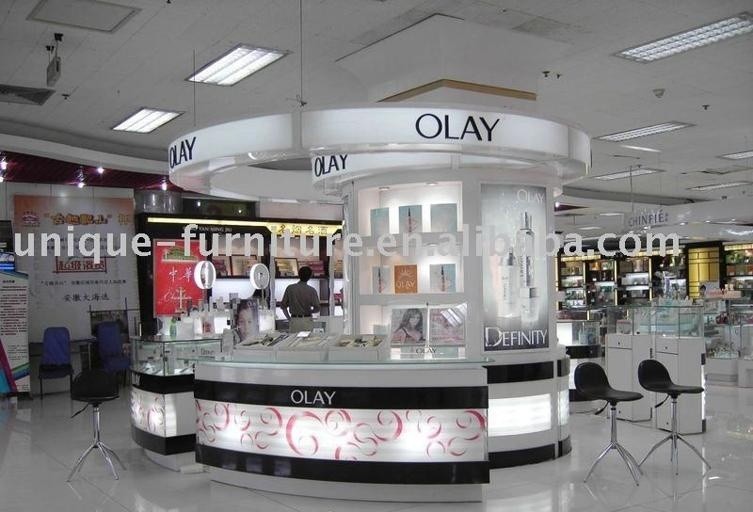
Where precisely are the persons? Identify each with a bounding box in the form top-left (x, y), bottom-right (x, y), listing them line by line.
top-left (700, 285), bottom-right (706, 296)
top-left (393, 309), bottom-right (425, 343)
top-left (281, 266), bottom-right (321, 332)
top-left (237, 302), bottom-right (253, 339)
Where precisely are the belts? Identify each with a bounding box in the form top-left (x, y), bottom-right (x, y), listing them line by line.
top-left (291, 315), bottom-right (311, 317)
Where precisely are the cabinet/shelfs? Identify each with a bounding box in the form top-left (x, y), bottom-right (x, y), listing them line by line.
top-left (556, 247), bottom-right (661, 311)
top-left (719, 242), bottom-right (752, 305)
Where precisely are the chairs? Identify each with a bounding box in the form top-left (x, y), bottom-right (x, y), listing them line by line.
top-left (35, 320), bottom-right (132, 483)
top-left (572, 357), bottom-right (712, 486)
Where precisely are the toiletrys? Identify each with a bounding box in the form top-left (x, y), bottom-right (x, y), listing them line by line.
top-left (499, 211), bottom-right (540, 320)
top-left (169, 297), bottom-right (217, 336)
